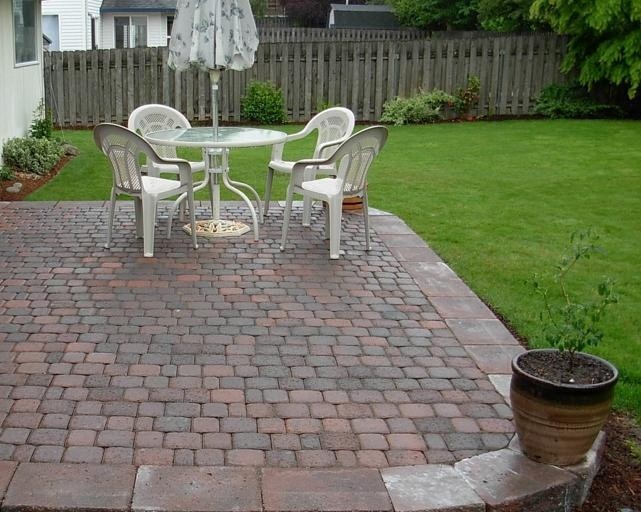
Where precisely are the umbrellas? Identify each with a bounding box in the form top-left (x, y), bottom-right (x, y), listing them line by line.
top-left (166, 0), bottom-right (260, 238)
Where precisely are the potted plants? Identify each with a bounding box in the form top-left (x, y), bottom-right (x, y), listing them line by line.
top-left (511, 230), bottom-right (627, 467)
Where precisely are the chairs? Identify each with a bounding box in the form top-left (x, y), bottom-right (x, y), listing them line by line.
top-left (127, 104), bottom-right (192, 219)
top-left (93, 123), bottom-right (199, 258)
top-left (281, 125), bottom-right (388, 260)
top-left (264, 107), bottom-right (355, 217)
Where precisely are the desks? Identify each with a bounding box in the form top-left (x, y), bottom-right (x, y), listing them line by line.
top-left (147, 126), bottom-right (285, 242)
